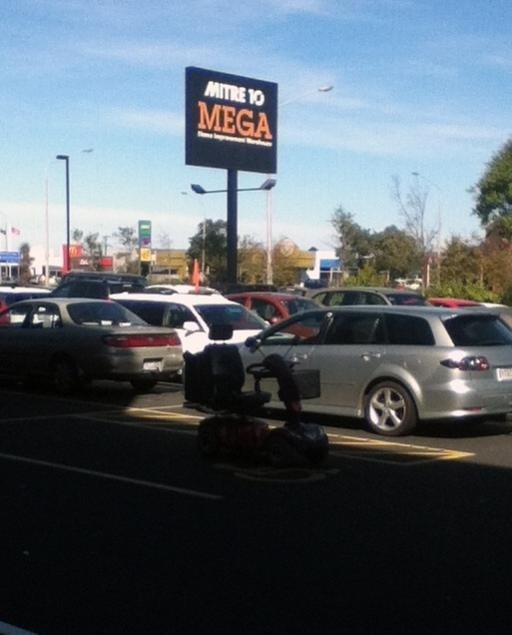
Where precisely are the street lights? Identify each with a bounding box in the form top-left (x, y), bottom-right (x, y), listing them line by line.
top-left (55, 153), bottom-right (72, 272)
top-left (44, 146), bottom-right (94, 286)
top-left (411, 170), bottom-right (443, 273)
top-left (189, 177), bottom-right (276, 283)
top-left (263, 84), bottom-right (333, 286)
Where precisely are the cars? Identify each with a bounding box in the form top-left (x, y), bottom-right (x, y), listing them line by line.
top-left (182, 306), bottom-right (512, 436)
top-left (0, 297), bottom-right (184, 392)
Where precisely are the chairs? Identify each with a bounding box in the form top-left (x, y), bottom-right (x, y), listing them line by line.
top-left (203, 345), bottom-right (270, 417)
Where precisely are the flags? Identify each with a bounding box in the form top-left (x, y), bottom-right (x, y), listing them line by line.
top-left (11, 227), bottom-right (20, 236)
top-left (0, 228), bottom-right (6, 235)
